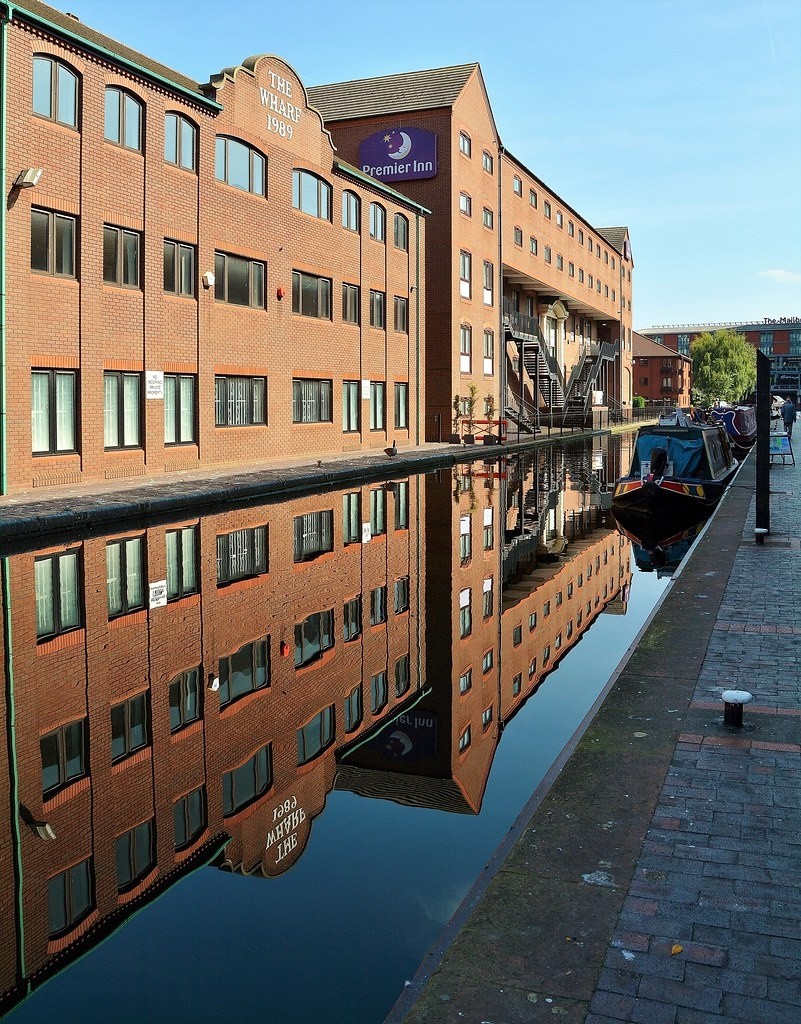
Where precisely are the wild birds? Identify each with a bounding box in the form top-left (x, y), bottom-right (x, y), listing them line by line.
top-left (382, 440), bottom-right (397, 461)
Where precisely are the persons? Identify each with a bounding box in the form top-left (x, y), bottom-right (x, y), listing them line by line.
top-left (781, 397), bottom-right (796, 439)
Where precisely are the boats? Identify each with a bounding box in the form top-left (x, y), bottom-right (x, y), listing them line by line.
top-left (610, 499), bottom-right (721, 577)
top-left (691, 404), bottom-right (756, 459)
top-left (613, 409), bottom-right (739, 506)
